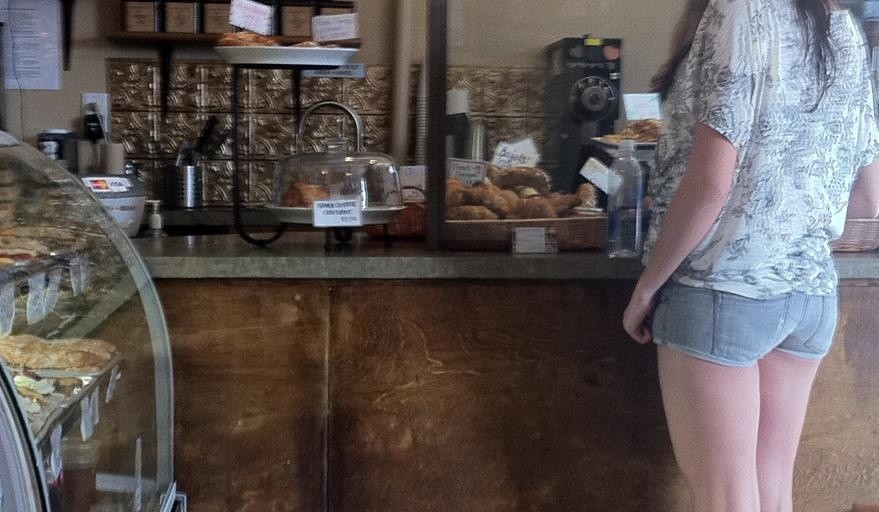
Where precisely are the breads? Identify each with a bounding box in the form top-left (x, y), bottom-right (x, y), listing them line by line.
top-left (282, 181), bottom-right (330, 207)
top-left (291, 41), bottom-right (337, 48)
top-left (445, 163), bottom-right (582, 219)
top-left (218, 30), bottom-right (280, 46)
top-left (0, 225), bottom-right (78, 265)
top-left (0, 334), bottom-right (117, 414)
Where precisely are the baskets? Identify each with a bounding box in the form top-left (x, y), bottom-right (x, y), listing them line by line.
top-left (366, 185), bottom-right (425, 240)
top-left (442, 213), bottom-right (607, 253)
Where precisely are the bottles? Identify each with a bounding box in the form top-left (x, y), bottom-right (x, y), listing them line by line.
top-left (74, 102), bottom-right (105, 176)
top-left (608, 138), bottom-right (645, 259)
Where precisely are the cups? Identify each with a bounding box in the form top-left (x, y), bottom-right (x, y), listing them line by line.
top-left (101, 142), bottom-right (124, 176)
top-left (164, 166), bottom-right (202, 211)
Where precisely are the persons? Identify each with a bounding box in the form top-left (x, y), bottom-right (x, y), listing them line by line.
top-left (622, 0), bottom-right (879, 512)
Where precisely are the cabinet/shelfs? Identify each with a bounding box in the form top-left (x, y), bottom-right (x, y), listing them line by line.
top-left (0, 130), bottom-right (189, 512)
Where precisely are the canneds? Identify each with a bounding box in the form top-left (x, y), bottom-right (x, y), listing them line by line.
top-left (36, 128), bottom-right (77, 173)
